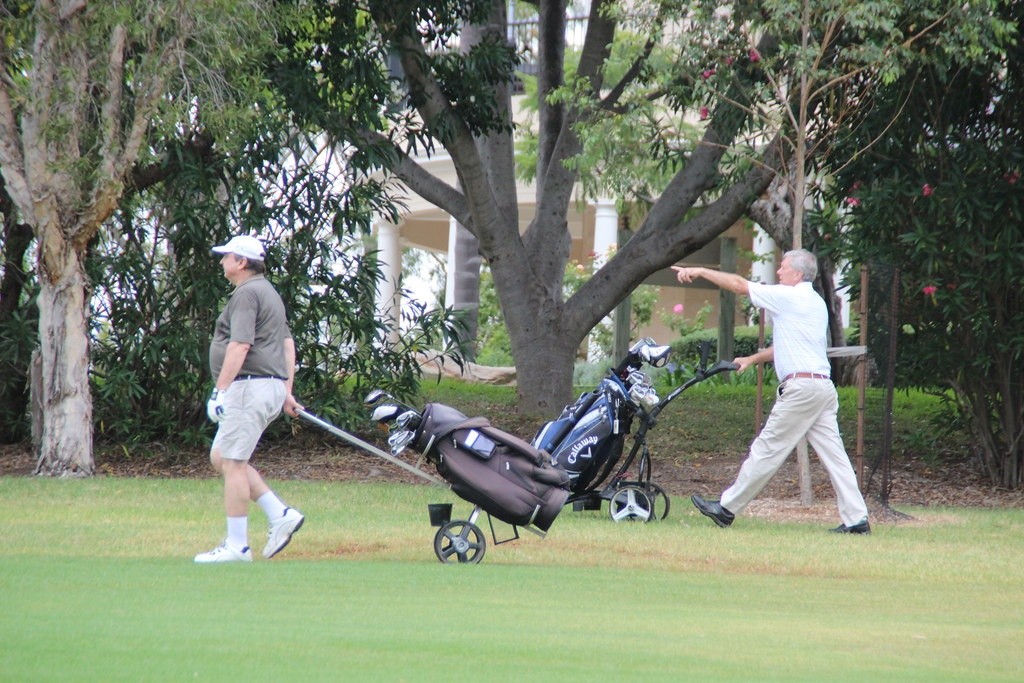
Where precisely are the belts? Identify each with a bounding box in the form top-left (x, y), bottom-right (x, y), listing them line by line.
top-left (234, 374), bottom-right (282, 381)
top-left (781, 372), bottom-right (828, 382)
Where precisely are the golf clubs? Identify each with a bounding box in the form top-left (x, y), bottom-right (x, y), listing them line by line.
top-left (618, 367), bottom-right (661, 406)
top-left (364, 390), bottom-right (421, 459)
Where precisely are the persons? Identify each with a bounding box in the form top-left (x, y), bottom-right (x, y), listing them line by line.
top-left (670, 248), bottom-right (873, 533)
top-left (194, 234), bottom-right (305, 565)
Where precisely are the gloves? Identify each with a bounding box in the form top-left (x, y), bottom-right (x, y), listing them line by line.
top-left (207, 387), bottom-right (226, 423)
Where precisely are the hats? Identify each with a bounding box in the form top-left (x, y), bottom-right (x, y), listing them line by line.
top-left (212, 236), bottom-right (264, 261)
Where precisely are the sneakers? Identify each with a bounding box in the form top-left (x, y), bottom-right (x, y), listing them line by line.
top-left (262, 508), bottom-right (304, 559)
top-left (828, 520), bottom-right (871, 537)
top-left (691, 495), bottom-right (735, 528)
top-left (195, 535), bottom-right (251, 564)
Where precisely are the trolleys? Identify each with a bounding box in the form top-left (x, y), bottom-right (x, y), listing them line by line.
top-left (295, 392), bottom-right (574, 567)
top-left (522, 338), bottom-right (741, 529)
